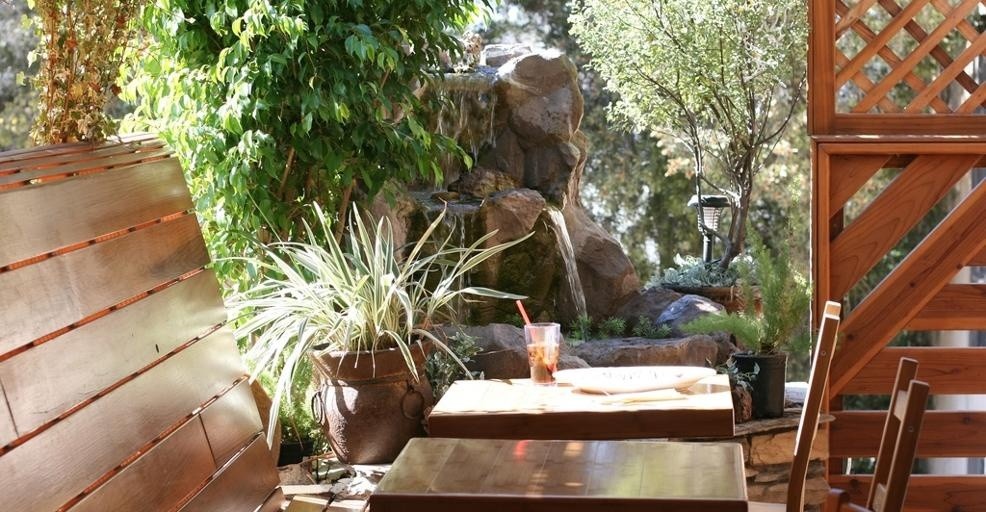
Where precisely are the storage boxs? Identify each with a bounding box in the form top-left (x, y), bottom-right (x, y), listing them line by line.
top-left (0, 135), bottom-right (373, 512)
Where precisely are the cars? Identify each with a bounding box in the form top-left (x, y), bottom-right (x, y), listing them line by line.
top-left (522, 321), bottom-right (562, 388)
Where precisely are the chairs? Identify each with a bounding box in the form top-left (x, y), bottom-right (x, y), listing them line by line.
top-left (751, 301), bottom-right (926, 511)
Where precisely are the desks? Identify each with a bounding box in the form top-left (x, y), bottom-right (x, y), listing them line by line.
top-left (367, 438), bottom-right (749, 511)
top-left (427, 373), bottom-right (735, 443)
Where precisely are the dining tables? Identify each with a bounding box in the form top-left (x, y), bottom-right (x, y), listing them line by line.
top-left (208, 196), bottom-right (534, 465)
top-left (675, 217), bottom-right (812, 419)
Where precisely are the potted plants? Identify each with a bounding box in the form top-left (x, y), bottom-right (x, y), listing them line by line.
top-left (675, 217), bottom-right (812, 419)
top-left (208, 196), bottom-right (534, 465)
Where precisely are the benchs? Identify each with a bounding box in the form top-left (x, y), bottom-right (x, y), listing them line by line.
top-left (0, 135), bottom-right (373, 512)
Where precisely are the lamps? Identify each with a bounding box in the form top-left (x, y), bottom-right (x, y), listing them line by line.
top-left (751, 301), bottom-right (926, 511)
